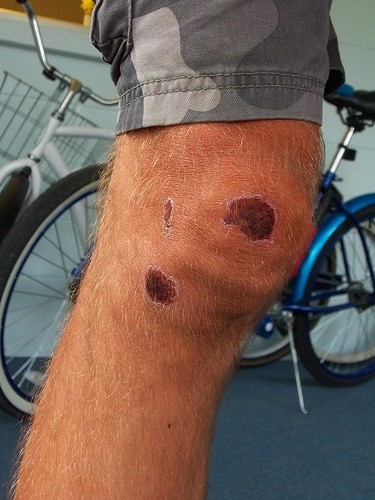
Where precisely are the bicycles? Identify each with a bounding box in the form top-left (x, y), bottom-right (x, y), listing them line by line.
top-left (1, 0), bottom-right (375, 424)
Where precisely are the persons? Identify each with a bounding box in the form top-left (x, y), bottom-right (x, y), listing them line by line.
top-left (7, 0), bottom-right (334, 499)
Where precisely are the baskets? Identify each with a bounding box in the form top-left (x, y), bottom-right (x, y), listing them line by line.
top-left (0, 72), bottom-right (110, 186)
